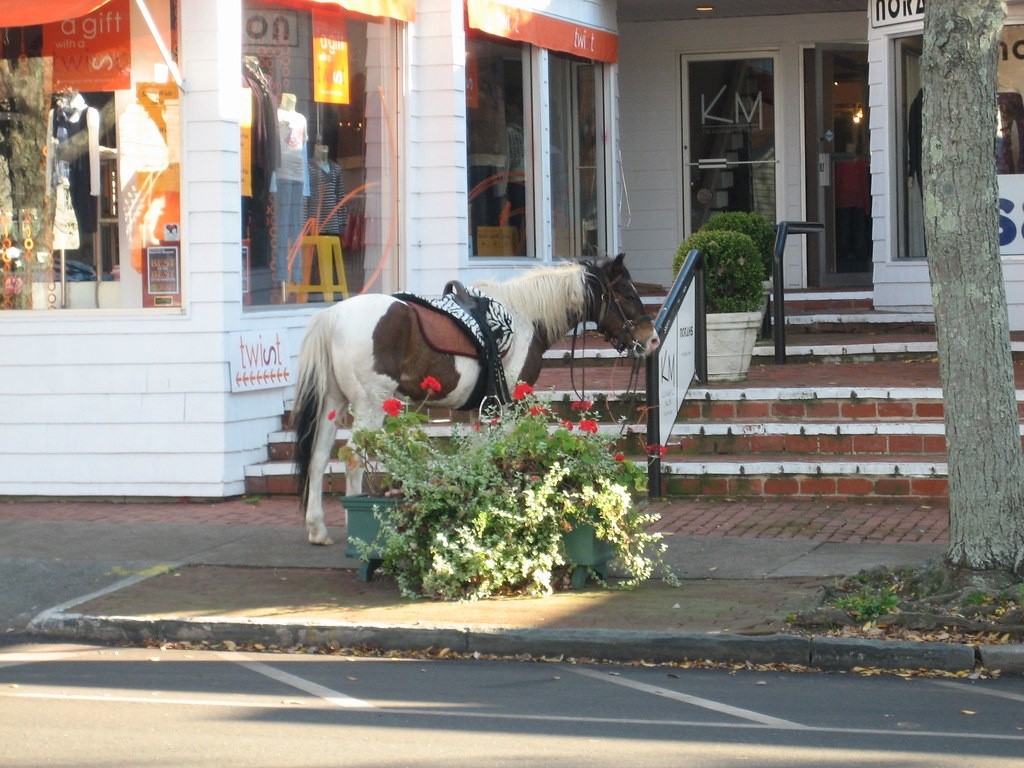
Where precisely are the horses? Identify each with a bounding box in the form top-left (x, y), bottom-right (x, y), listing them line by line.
top-left (289, 251), bottom-right (662, 544)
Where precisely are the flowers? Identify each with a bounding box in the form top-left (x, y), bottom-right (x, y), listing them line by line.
top-left (312, 376), bottom-right (680, 604)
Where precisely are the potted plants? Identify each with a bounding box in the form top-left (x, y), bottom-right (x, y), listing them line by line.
top-left (672, 212), bottom-right (775, 381)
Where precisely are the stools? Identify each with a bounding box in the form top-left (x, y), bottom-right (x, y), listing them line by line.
top-left (280, 236), bottom-right (349, 303)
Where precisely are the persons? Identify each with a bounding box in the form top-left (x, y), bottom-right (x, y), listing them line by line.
top-left (493, 99), bottom-right (524, 233)
top-left (833, 142), bottom-right (871, 259)
top-left (40, 85), bottom-right (102, 237)
top-left (243, 53), bottom-right (348, 286)
top-left (903, 73), bottom-right (1024, 257)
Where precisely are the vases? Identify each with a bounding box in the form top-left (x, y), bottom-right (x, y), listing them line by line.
top-left (340, 491), bottom-right (612, 589)
